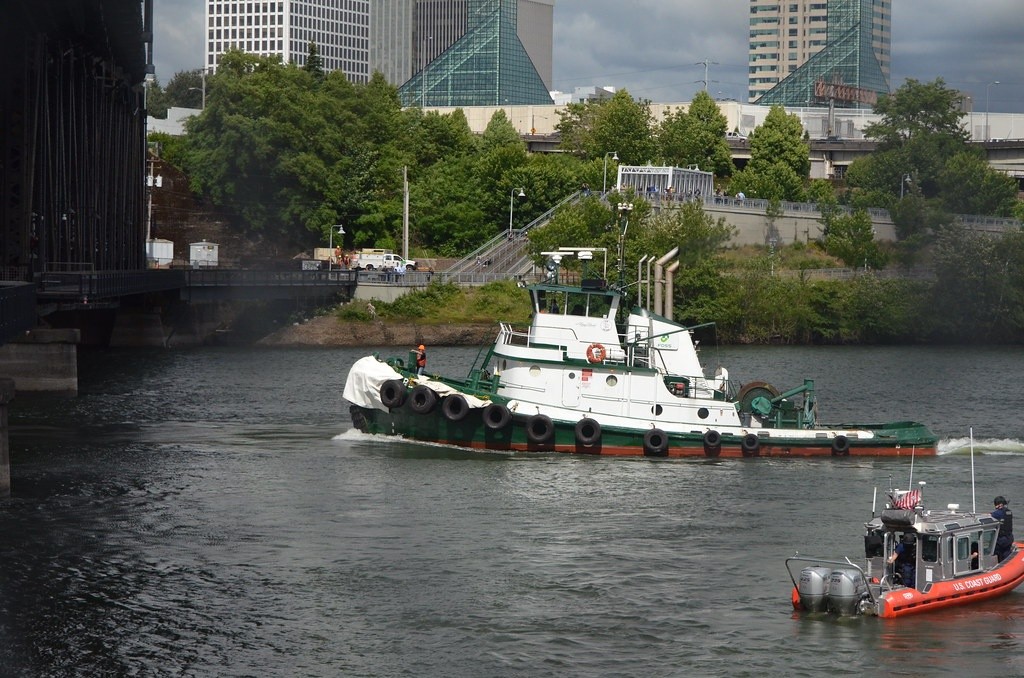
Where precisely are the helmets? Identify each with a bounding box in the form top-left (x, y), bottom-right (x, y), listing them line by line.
top-left (418, 345), bottom-right (425, 351)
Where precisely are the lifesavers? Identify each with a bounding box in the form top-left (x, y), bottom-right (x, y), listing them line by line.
top-left (409, 385), bottom-right (438, 414)
top-left (442, 394), bottom-right (469, 422)
top-left (704, 430), bottom-right (720, 447)
top-left (575, 418), bottom-right (602, 444)
top-left (526, 414), bottom-right (554, 443)
top-left (587, 343), bottom-right (607, 363)
top-left (380, 380), bottom-right (407, 408)
top-left (742, 433), bottom-right (759, 450)
top-left (483, 404), bottom-right (511, 431)
top-left (643, 428), bottom-right (667, 452)
top-left (832, 435), bottom-right (849, 452)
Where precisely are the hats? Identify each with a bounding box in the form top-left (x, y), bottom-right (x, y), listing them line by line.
top-left (337, 245), bottom-right (340, 249)
top-left (339, 255), bottom-right (342, 258)
top-left (902, 533), bottom-right (914, 546)
top-left (346, 254), bottom-right (348, 257)
top-left (994, 496), bottom-right (1007, 505)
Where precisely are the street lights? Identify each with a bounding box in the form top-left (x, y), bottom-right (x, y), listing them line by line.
top-left (901, 173), bottom-right (912, 198)
top-left (986, 80), bottom-right (1001, 142)
top-left (509, 187), bottom-right (525, 231)
top-left (603, 152), bottom-right (620, 194)
top-left (421, 37), bottom-right (434, 106)
top-left (328, 224), bottom-right (346, 270)
top-left (967, 96), bottom-right (973, 140)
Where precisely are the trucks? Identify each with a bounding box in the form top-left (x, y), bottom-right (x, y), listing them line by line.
top-left (350, 254), bottom-right (417, 271)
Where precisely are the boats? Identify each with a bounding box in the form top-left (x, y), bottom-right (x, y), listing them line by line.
top-left (786, 425), bottom-right (1024, 618)
top-left (343, 201), bottom-right (940, 460)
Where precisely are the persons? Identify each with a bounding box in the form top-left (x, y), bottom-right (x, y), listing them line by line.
top-left (355, 263), bottom-right (361, 280)
top-left (328, 246), bottom-right (350, 266)
top-left (508, 233), bottom-right (514, 241)
top-left (549, 299), bottom-right (559, 314)
top-left (412, 345), bottom-right (426, 374)
top-left (429, 265), bottom-right (433, 272)
top-left (483, 255), bottom-right (492, 267)
top-left (887, 533), bottom-right (916, 588)
top-left (694, 189), bottom-right (701, 198)
top-left (990, 496), bottom-right (1014, 563)
top-left (612, 182), bottom-right (627, 192)
top-left (581, 183), bottom-right (590, 196)
top-left (737, 191), bottom-right (745, 203)
top-left (638, 185), bottom-right (672, 199)
